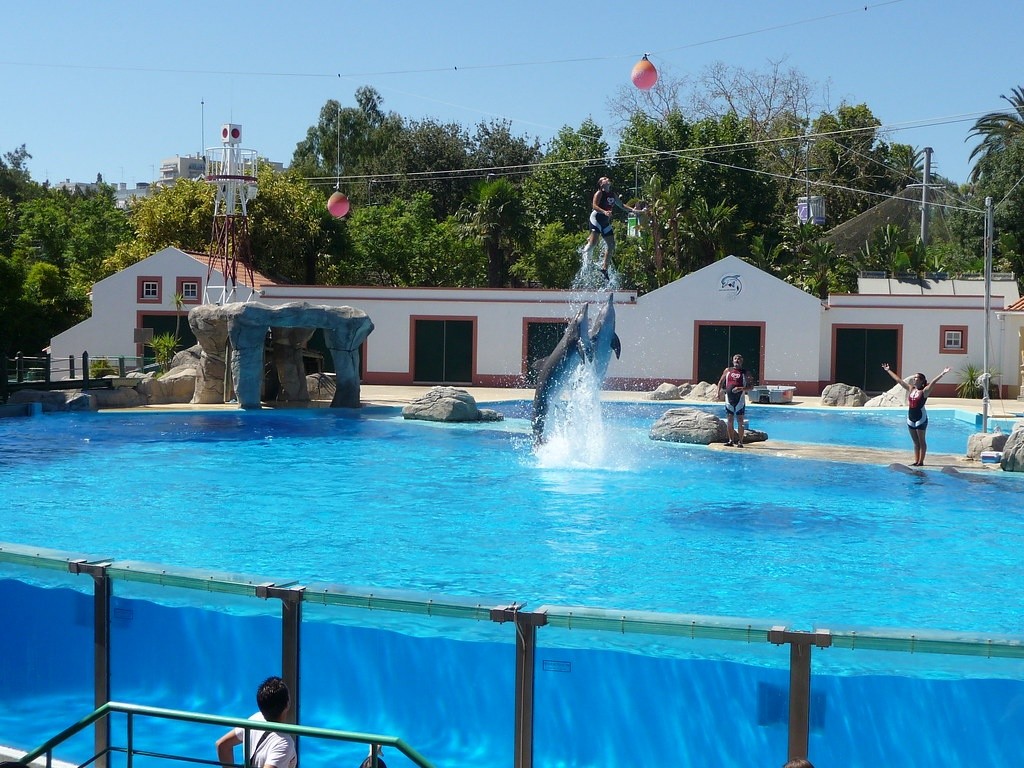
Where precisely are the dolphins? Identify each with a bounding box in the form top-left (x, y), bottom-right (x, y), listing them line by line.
top-left (531, 292), bottom-right (621, 448)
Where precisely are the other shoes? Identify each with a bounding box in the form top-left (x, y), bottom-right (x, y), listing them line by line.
top-left (909, 462), bottom-right (917, 465)
top-left (916, 462), bottom-right (923, 466)
top-left (724, 441), bottom-right (734, 446)
top-left (738, 442), bottom-right (743, 448)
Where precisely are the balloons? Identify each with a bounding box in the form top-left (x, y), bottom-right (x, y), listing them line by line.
top-left (327, 191), bottom-right (350, 219)
top-left (632, 59), bottom-right (657, 91)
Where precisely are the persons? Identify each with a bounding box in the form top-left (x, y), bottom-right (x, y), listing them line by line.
top-left (881, 363), bottom-right (951, 466)
top-left (584, 177), bottom-right (637, 278)
top-left (360, 756), bottom-right (387, 768)
top-left (216, 675), bottom-right (298, 768)
top-left (715, 354), bottom-right (754, 447)
